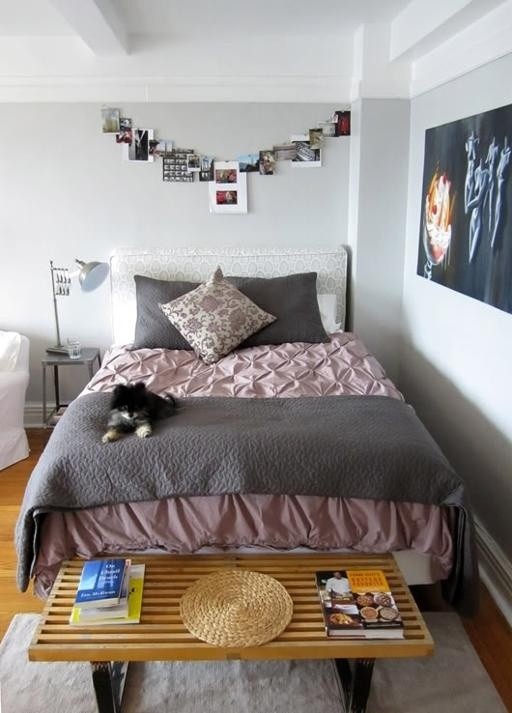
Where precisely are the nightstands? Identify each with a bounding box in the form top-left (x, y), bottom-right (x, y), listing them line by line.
top-left (40, 347), bottom-right (102, 430)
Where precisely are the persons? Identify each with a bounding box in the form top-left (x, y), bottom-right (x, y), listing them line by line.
top-left (325, 572), bottom-right (351, 598)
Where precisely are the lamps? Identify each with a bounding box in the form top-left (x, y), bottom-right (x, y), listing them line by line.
top-left (46, 257), bottom-right (110, 355)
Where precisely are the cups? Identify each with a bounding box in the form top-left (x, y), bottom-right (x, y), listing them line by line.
top-left (67, 340), bottom-right (81, 360)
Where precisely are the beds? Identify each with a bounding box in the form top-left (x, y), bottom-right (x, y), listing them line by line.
top-left (13, 242), bottom-right (478, 619)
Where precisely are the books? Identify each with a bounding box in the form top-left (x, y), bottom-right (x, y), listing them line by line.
top-left (314, 570), bottom-right (406, 638)
top-left (70, 557), bottom-right (145, 627)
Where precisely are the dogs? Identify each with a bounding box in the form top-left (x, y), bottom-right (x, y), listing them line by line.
top-left (100, 381), bottom-right (182, 443)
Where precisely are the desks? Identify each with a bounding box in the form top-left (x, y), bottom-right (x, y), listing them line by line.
top-left (26, 553), bottom-right (435, 713)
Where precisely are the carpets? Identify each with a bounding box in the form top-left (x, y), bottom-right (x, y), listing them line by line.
top-left (1, 612), bottom-right (508, 712)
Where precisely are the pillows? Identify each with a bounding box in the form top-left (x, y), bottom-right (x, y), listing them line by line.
top-left (158, 263), bottom-right (278, 366)
top-left (125, 273), bottom-right (332, 352)
